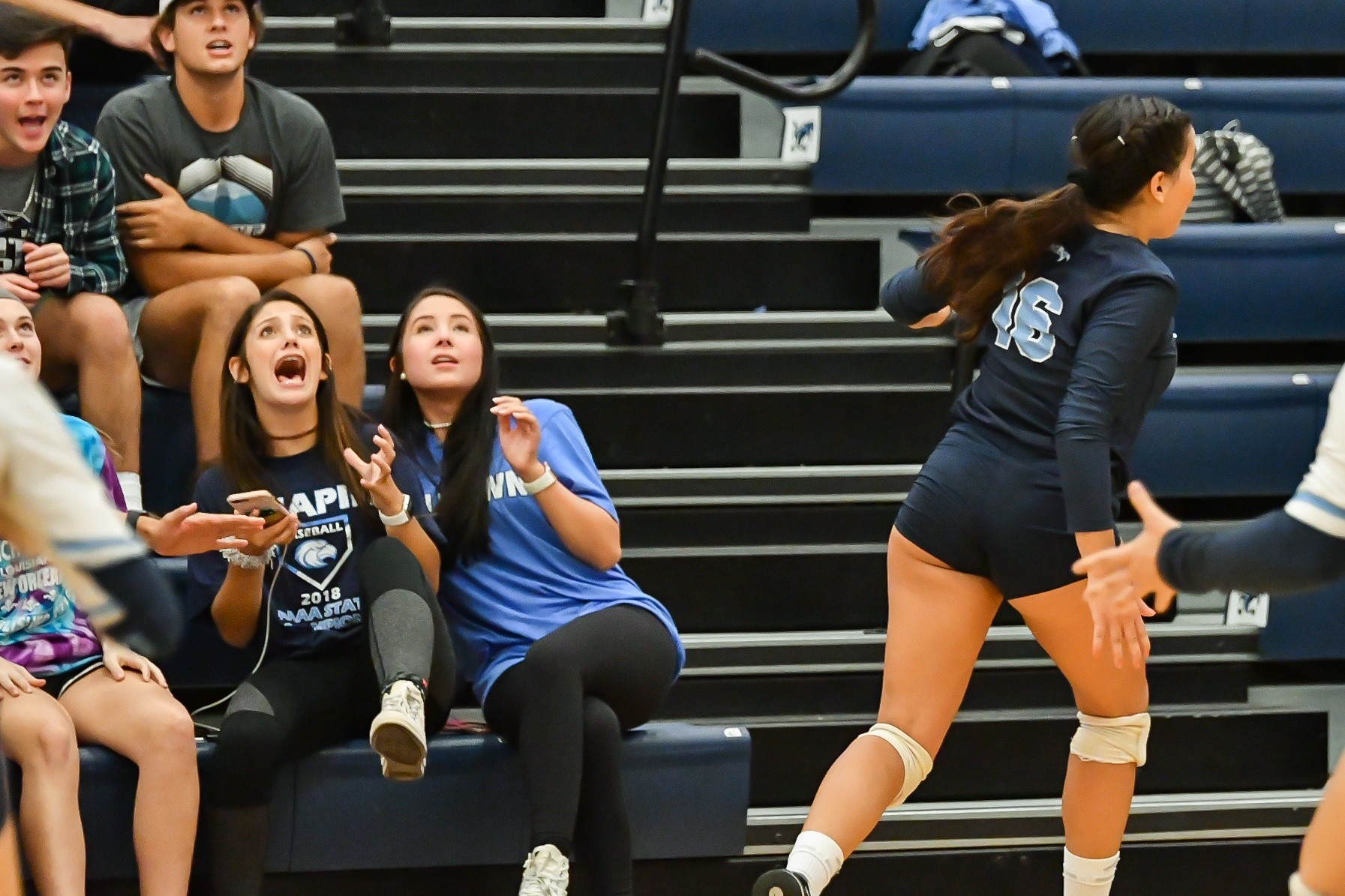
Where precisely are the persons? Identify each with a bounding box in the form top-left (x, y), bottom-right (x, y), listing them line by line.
top-left (0, 0), bottom-right (457, 896)
top-left (384, 287), bottom-right (687, 896)
top-left (1071, 364), bottom-right (1345, 896)
top-left (749, 93), bottom-right (1196, 896)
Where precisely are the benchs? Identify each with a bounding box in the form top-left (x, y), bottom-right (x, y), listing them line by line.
top-left (0, 0), bottom-right (1345, 896)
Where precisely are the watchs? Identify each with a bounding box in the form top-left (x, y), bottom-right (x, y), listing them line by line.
top-left (126, 509), bottom-right (150, 528)
top-left (378, 492), bottom-right (415, 529)
top-left (524, 461), bottom-right (557, 495)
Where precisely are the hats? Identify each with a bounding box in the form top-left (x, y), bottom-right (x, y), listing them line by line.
top-left (149, 0), bottom-right (266, 73)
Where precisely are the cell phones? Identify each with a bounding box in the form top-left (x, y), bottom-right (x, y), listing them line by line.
top-left (227, 488), bottom-right (303, 528)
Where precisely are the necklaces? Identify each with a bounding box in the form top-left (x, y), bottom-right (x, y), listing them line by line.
top-left (422, 419), bottom-right (452, 430)
top-left (268, 423), bottom-right (317, 440)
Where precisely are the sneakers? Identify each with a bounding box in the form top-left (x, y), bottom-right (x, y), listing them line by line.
top-left (370, 681), bottom-right (428, 782)
top-left (519, 844), bottom-right (570, 896)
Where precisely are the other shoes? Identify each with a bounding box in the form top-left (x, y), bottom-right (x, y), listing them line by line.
top-left (752, 868), bottom-right (814, 896)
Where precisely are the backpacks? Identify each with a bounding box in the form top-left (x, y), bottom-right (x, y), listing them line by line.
top-left (1181, 119), bottom-right (1286, 224)
top-left (908, 0), bottom-right (1090, 78)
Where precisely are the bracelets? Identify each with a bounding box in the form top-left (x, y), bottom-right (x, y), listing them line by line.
top-left (297, 248), bottom-right (317, 274)
top-left (218, 536), bottom-right (277, 568)
